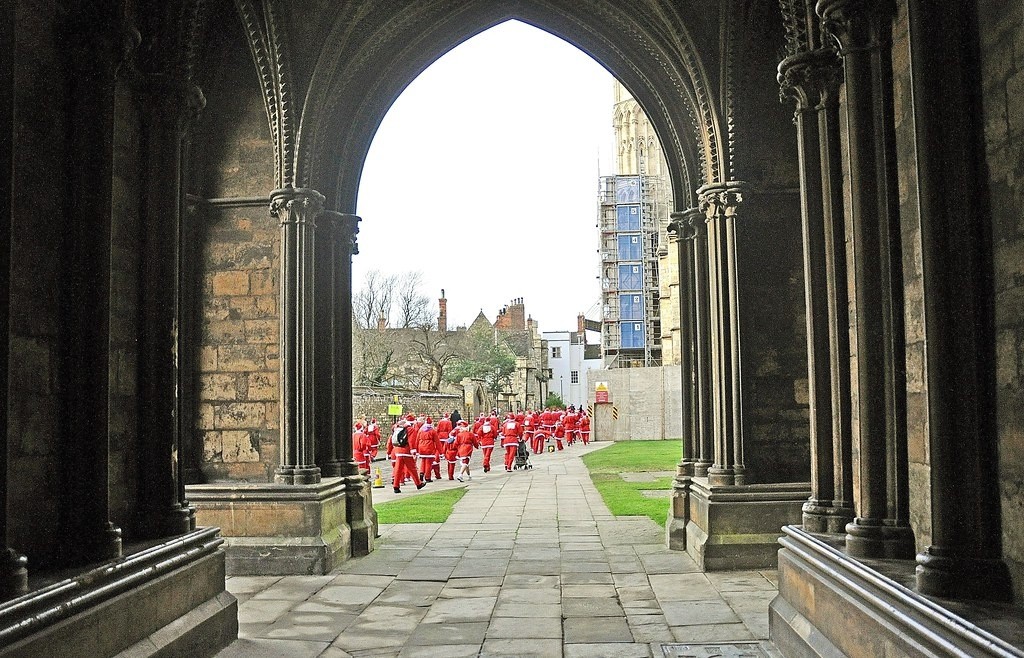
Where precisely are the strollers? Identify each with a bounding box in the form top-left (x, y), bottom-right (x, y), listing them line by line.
top-left (513, 436), bottom-right (533, 470)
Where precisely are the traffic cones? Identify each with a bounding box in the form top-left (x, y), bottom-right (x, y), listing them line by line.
top-left (373, 466), bottom-right (385, 488)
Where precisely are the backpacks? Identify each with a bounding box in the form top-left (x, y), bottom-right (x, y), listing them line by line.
top-left (392, 425), bottom-right (411, 447)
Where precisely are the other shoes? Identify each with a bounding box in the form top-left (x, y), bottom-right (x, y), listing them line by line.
top-left (400, 483), bottom-right (406, 486)
top-left (466, 477), bottom-right (471, 481)
top-left (437, 477), bottom-right (441, 479)
top-left (417, 482), bottom-right (426, 489)
top-left (484, 465), bottom-right (487, 473)
top-left (420, 474), bottom-right (423, 481)
top-left (507, 471), bottom-right (513, 472)
top-left (426, 479), bottom-right (433, 482)
top-left (449, 478), bottom-right (454, 480)
top-left (404, 478), bottom-right (411, 482)
top-left (505, 466), bottom-right (507, 470)
top-left (394, 488), bottom-right (401, 493)
top-left (457, 476), bottom-right (464, 482)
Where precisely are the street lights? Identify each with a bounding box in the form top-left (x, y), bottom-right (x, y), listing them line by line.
top-left (560, 375), bottom-right (564, 405)
top-left (494, 363), bottom-right (502, 415)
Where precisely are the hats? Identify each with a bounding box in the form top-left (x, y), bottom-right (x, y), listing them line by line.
top-left (355, 407), bottom-right (586, 431)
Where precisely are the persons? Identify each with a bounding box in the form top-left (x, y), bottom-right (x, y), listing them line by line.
top-left (352, 403), bottom-right (591, 494)
